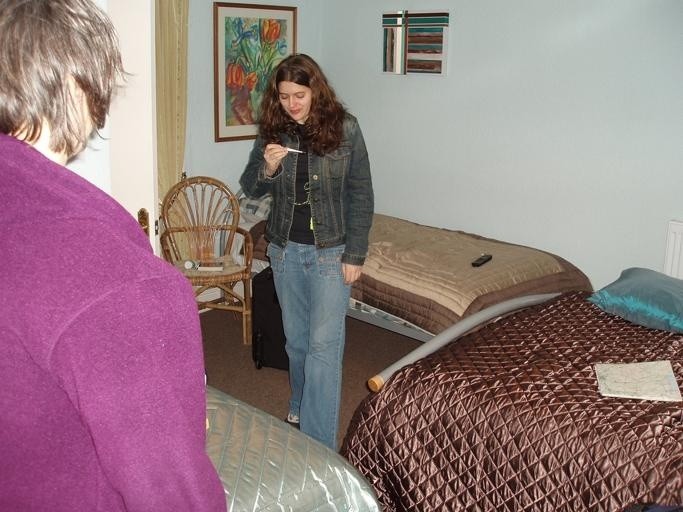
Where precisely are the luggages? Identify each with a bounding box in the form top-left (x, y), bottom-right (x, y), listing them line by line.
top-left (250, 264), bottom-right (290, 372)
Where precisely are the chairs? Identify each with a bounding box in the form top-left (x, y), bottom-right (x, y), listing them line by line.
top-left (159, 174), bottom-right (256, 348)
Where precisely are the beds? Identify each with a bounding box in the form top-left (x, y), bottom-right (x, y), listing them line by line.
top-left (196, 375), bottom-right (387, 512)
top-left (214, 182), bottom-right (595, 346)
top-left (334, 288), bottom-right (682, 512)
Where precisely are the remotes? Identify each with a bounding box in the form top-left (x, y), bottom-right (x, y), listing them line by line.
top-left (471, 254), bottom-right (492, 266)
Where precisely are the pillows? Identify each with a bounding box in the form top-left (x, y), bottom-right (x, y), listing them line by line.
top-left (220, 190), bottom-right (273, 226)
top-left (583, 265), bottom-right (682, 336)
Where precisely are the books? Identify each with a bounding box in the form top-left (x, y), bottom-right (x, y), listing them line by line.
top-left (197, 261), bottom-right (223, 272)
top-left (595, 360), bottom-right (683, 401)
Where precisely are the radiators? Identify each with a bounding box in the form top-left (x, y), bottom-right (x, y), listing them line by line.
top-left (662, 218), bottom-right (683, 283)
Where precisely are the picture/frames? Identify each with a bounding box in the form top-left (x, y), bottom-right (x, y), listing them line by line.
top-left (209, 1), bottom-right (298, 144)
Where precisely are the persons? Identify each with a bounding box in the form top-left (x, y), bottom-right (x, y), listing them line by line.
top-left (0, 0), bottom-right (227, 512)
top-left (239, 54), bottom-right (374, 451)
